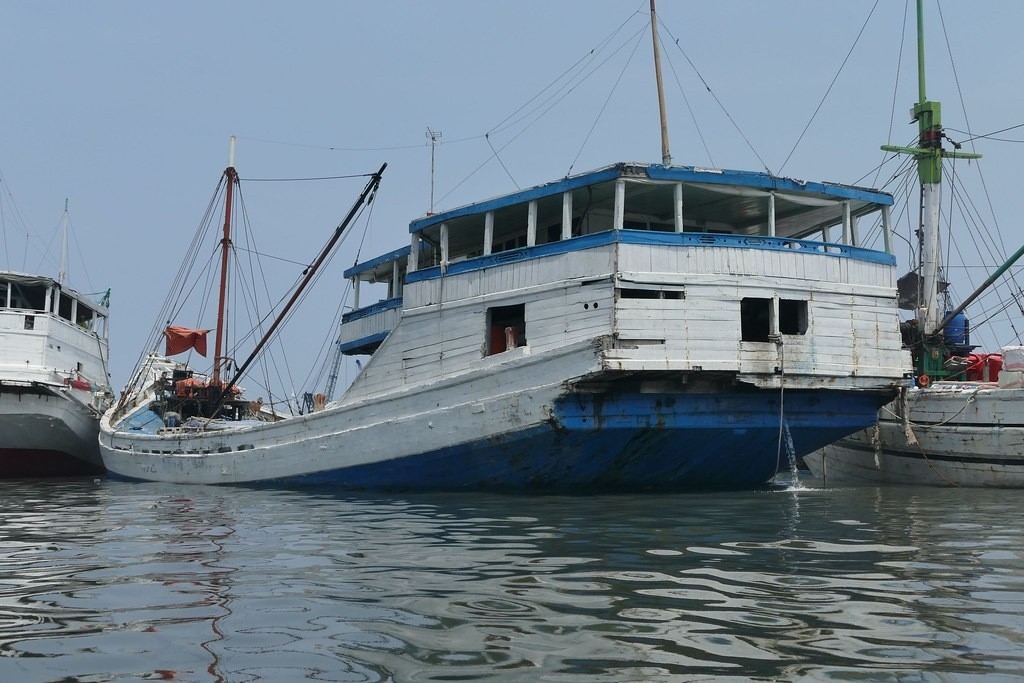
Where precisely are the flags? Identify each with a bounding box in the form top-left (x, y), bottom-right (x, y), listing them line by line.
top-left (162, 327), bottom-right (212, 358)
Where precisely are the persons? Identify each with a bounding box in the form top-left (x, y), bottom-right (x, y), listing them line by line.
top-left (76, 316), bottom-right (88, 329)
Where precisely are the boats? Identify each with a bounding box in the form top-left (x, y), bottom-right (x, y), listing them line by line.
top-left (802, 1), bottom-right (1023, 490)
top-left (0, 172), bottom-right (110, 468)
top-left (99, 1), bottom-right (918, 499)
